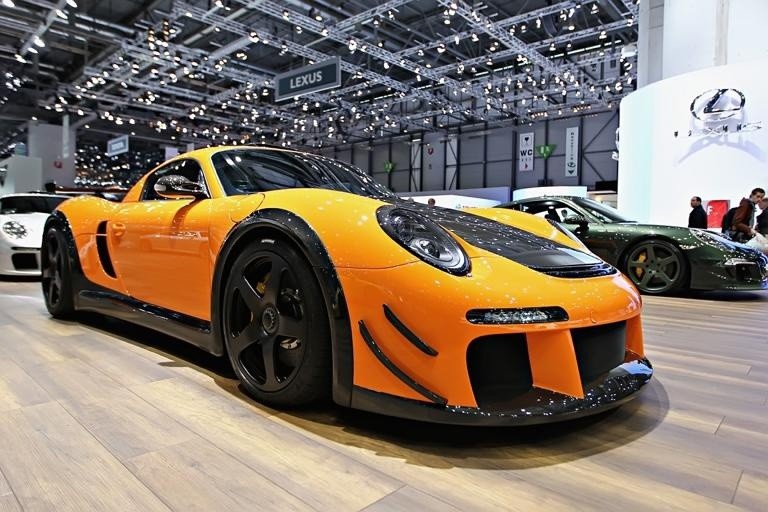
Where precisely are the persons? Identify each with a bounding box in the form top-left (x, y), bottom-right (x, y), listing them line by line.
top-left (755, 198), bottom-right (768, 234)
top-left (561, 210), bottom-right (569, 222)
top-left (731, 188), bottom-right (765, 236)
top-left (688, 197), bottom-right (707, 228)
top-left (428, 199), bottom-right (435, 205)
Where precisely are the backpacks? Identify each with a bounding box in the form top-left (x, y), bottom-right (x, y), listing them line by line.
top-left (721, 207), bottom-right (739, 234)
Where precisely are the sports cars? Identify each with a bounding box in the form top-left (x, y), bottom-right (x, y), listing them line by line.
top-left (41, 145), bottom-right (652, 427)
top-left (1, 193), bottom-right (74, 278)
top-left (492, 195), bottom-right (766, 296)
